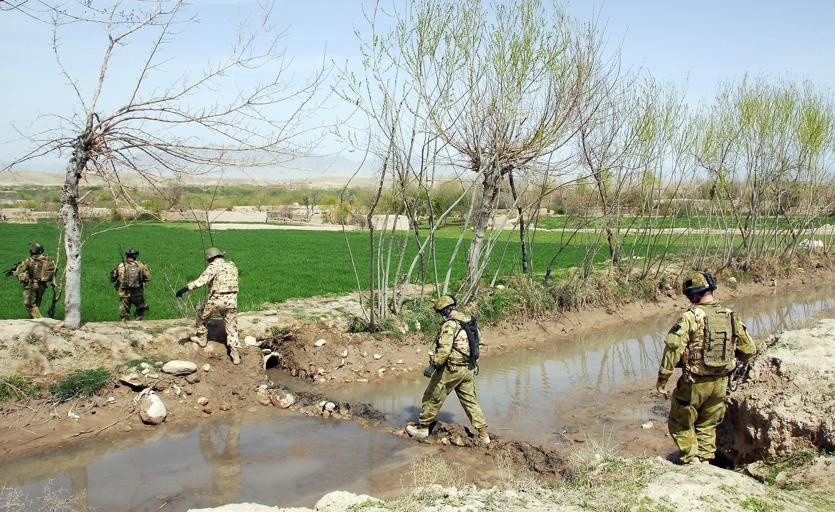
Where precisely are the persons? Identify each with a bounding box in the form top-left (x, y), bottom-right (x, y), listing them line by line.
top-left (11, 241), bottom-right (58, 318)
top-left (170, 420), bottom-right (244, 506)
top-left (655, 271), bottom-right (757, 467)
top-left (404, 294), bottom-right (491, 447)
top-left (108, 247), bottom-right (152, 320)
top-left (175, 246), bottom-right (241, 365)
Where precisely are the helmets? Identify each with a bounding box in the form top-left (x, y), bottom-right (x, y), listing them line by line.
top-left (681, 270), bottom-right (718, 295)
top-left (29, 242), bottom-right (44, 255)
top-left (124, 248), bottom-right (139, 258)
top-left (432, 294), bottom-right (458, 313)
top-left (204, 247), bottom-right (224, 261)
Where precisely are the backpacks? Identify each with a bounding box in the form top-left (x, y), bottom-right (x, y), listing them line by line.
top-left (32, 257), bottom-right (56, 282)
top-left (124, 260), bottom-right (144, 290)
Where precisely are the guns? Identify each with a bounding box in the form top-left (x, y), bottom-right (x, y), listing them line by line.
top-left (423, 328), bottom-right (441, 378)
top-left (3, 262), bottom-right (23, 277)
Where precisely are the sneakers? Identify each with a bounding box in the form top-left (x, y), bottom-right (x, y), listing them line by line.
top-left (189, 333), bottom-right (208, 348)
top-left (405, 424), bottom-right (430, 439)
top-left (230, 351), bottom-right (241, 365)
top-left (477, 432), bottom-right (491, 445)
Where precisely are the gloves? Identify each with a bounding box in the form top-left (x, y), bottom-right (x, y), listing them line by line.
top-left (423, 364), bottom-right (436, 379)
top-left (175, 286), bottom-right (188, 298)
